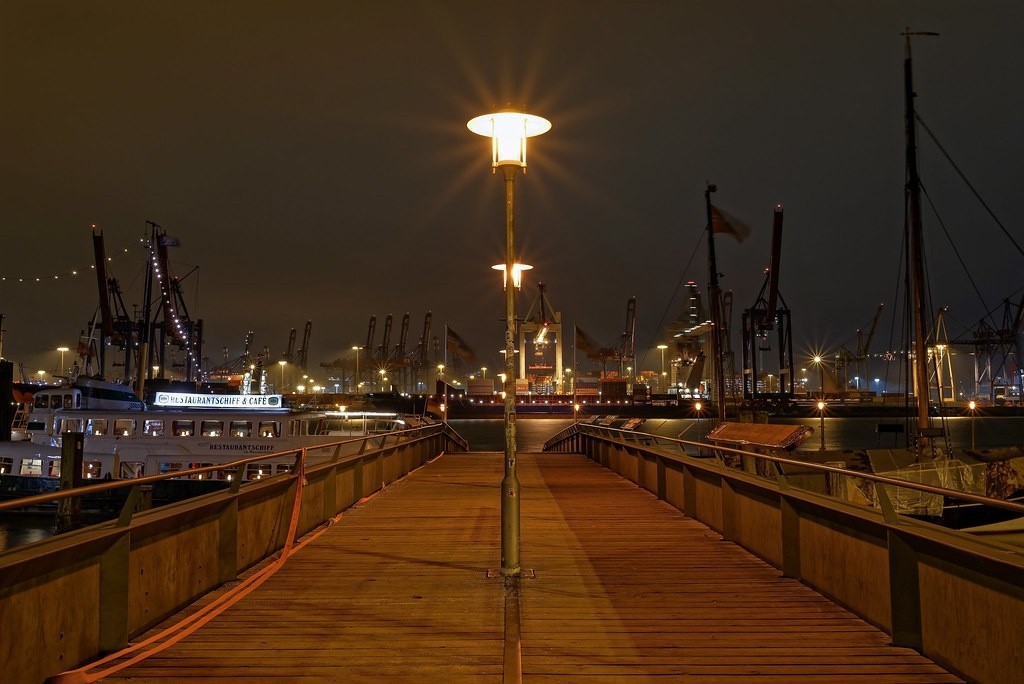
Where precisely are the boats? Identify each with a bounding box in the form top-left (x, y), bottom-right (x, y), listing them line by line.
top-left (0, 221), bottom-right (405, 483)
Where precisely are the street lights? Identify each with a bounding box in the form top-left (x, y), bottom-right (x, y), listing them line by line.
top-left (657, 344), bottom-right (667, 377)
top-left (695, 402), bottom-right (702, 457)
top-left (436, 365), bottom-right (445, 381)
top-left (492, 262), bottom-right (534, 292)
top-left (814, 354), bottom-right (827, 450)
top-left (768, 374), bottom-right (773, 394)
top-left (378, 370), bottom-right (386, 391)
top-left (278, 361), bottom-right (287, 394)
top-left (481, 367), bottom-right (488, 379)
top-left (466, 106), bottom-right (553, 578)
top-left (56, 347), bottom-right (69, 377)
top-left (351, 346), bottom-right (360, 394)
